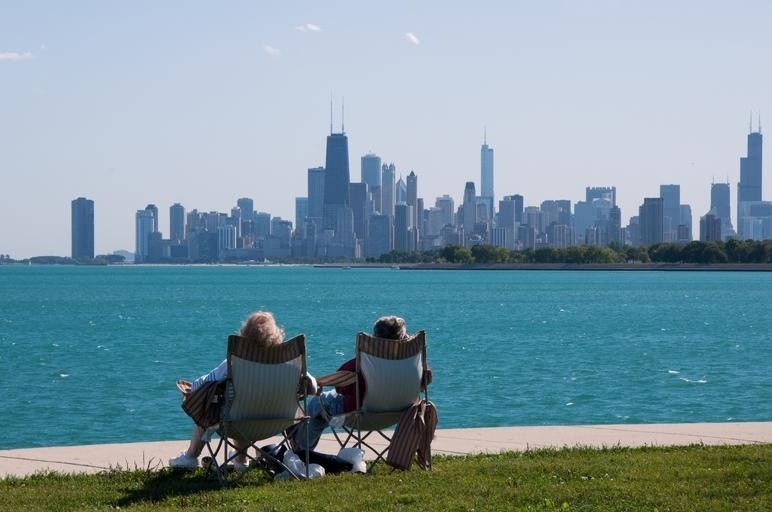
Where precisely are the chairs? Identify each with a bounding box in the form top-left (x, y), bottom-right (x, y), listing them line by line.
top-left (177, 333), bottom-right (311, 485)
top-left (315, 330), bottom-right (431, 475)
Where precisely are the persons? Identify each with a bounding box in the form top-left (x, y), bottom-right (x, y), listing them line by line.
top-left (287, 315), bottom-right (433, 455)
top-left (168, 310), bottom-right (319, 476)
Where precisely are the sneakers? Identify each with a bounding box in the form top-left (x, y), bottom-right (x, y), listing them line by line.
top-left (229, 451), bottom-right (247, 464)
top-left (169, 451), bottom-right (196, 467)
